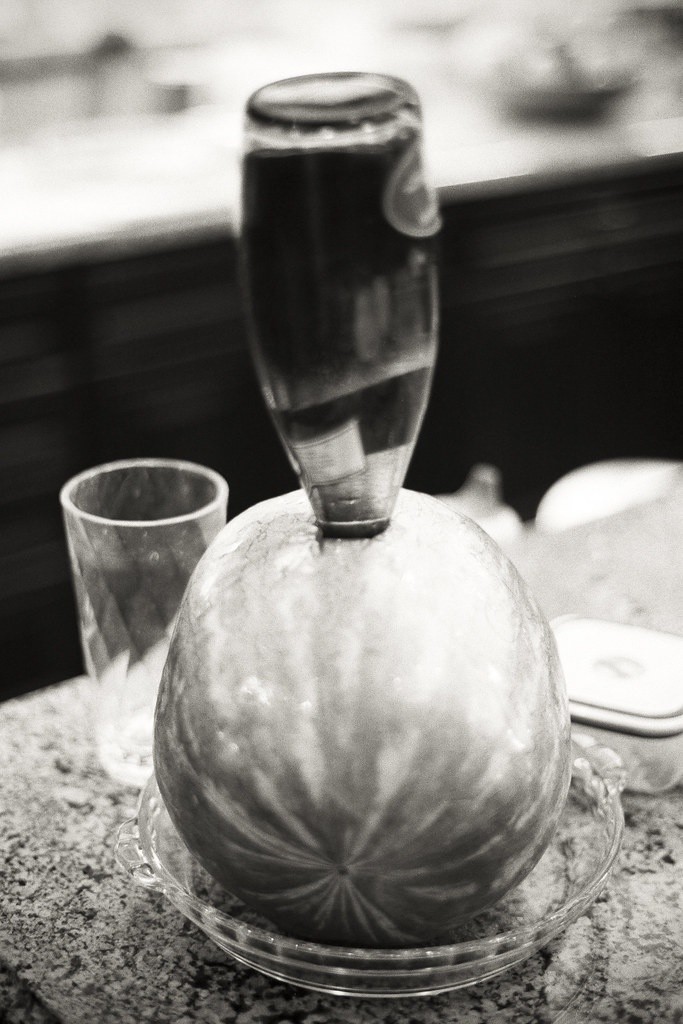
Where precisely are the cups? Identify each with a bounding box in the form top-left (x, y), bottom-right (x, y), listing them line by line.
top-left (56, 459), bottom-right (226, 780)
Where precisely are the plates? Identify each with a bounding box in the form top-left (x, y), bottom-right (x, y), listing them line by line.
top-left (114, 733), bottom-right (626, 997)
